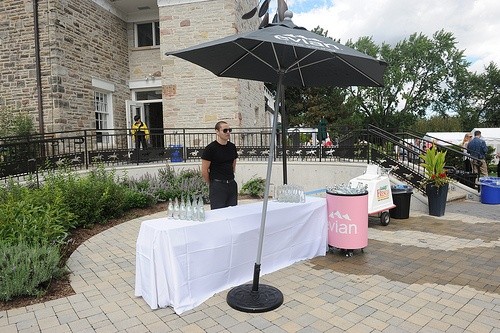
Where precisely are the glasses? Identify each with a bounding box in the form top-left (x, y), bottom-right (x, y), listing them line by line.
top-left (218, 127), bottom-right (232, 133)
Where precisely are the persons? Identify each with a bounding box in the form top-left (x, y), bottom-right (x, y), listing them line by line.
top-left (467, 130), bottom-right (488, 177)
top-left (398, 137), bottom-right (432, 162)
top-left (462, 132), bottom-right (473, 172)
top-left (201, 120), bottom-right (238, 210)
top-left (131, 115), bottom-right (150, 150)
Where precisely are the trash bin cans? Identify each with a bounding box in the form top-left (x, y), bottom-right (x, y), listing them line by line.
top-left (389, 185), bottom-right (413, 219)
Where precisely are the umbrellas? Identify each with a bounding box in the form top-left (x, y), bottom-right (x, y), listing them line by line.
top-left (317, 115), bottom-right (328, 162)
top-left (164, 10), bottom-right (389, 292)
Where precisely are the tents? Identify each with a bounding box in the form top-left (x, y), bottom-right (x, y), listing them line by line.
top-left (471, 128), bottom-right (500, 154)
top-left (422, 131), bottom-right (472, 147)
top-left (395, 138), bottom-right (422, 155)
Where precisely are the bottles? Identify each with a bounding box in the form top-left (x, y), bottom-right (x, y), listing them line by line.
top-left (326, 181), bottom-right (368, 194)
top-left (167, 193), bottom-right (205, 222)
top-left (273, 184), bottom-right (305, 203)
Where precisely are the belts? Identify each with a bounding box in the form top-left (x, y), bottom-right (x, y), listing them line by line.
top-left (213, 178), bottom-right (234, 183)
